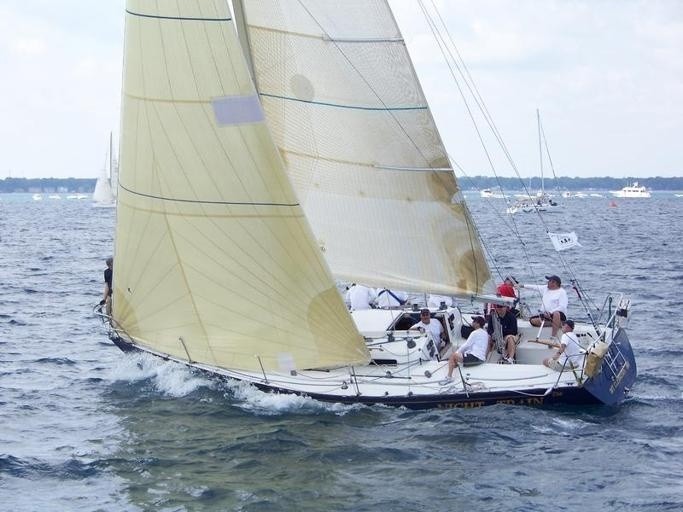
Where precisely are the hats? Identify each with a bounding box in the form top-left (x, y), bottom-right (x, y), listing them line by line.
top-left (563, 320), bottom-right (574, 329)
top-left (471, 316), bottom-right (484, 326)
top-left (505, 276), bottom-right (518, 284)
top-left (545, 275), bottom-right (561, 284)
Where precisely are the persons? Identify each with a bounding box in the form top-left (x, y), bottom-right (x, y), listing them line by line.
top-left (514, 276), bottom-right (568, 348)
top-left (543, 320), bottom-right (580, 372)
top-left (408, 309), bottom-right (446, 352)
top-left (486, 275), bottom-right (520, 364)
top-left (345, 282), bottom-right (408, 311)
top-left (437, 316), bottom-right (488, 387)
top-left (100, 257), bottom-right (112, 315)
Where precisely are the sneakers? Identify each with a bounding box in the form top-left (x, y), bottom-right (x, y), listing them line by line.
top-left (503, 357), bottom-right (514, 363)
top-left (440, 376), bottom-right (453, 384)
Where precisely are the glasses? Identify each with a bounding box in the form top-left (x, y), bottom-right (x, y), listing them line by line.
top-left (497, 304), bottom-right (506, 308)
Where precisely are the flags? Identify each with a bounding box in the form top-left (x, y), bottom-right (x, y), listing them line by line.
top-left (549, 232), bottom-right (583, 251)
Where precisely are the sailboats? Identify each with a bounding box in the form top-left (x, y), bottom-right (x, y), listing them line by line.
top-left (507, 109), bottom-right (568, 214)
top-left (92, 0), bottom-right (636, 410)
top-left (93, 132), bottom-right (118, 208)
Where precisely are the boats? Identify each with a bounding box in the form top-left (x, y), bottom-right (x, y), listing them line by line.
top-left (609, 182), bottom-right (652, 197)
